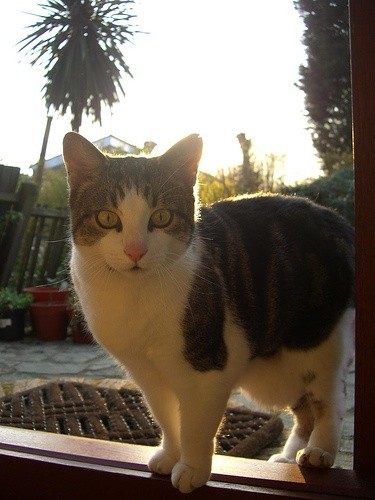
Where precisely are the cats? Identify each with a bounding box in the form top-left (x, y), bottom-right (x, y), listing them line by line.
top-left (62, 132), bottom-right (357, 496)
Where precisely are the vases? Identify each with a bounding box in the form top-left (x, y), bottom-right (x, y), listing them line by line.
top-left (25, 286), bottom-right (74, 341)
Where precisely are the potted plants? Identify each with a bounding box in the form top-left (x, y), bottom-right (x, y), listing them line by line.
top-left (7, 290), bottom-right (32, 340)
